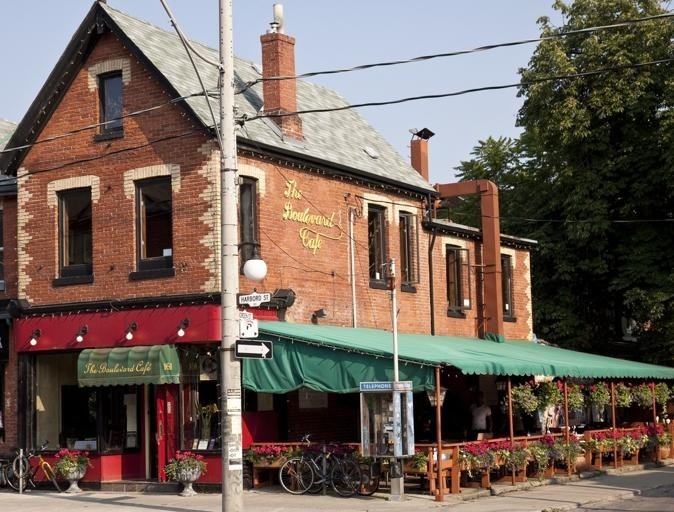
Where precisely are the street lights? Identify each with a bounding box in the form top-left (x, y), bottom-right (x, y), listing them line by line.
top-left (222, 241), bottom-right (268, 511)
top-left (375, 259), bottom-right (403, 494)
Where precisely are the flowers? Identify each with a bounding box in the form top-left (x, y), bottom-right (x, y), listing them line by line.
top-left (506, 383), bottom-right (584, 413)
top-left (613, 382), bottom-right (674, 414)
top-left (251, 445), bottom-right (294, 464)
top-left (407, 449), bottom-right (428, 468)
top-left (330, 444), bottom-right (366, 463)
top-left (52, 449), bottom-right (95, 479)
top-left (163, 451), bottom-right (208, 483)
top-left (589, 382), bottom-right (612, 403)
top-left (459, 434), bottom-right (569, 478)
top-left (569, 416), bottom-right (673, 451)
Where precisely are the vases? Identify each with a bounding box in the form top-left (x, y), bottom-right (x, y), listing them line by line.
top-left (591, 404), bottom-right (605, 422)
top-left (569, 452), bottom-right (586, 472)
top-left (660, 444), bottom-right (671, 459)
top-left (176, 471), bottom-right (202, 497)
top-left (536, 403), bottom-right (563, 428)
top-left (61, 472), bottom-right (86, 493)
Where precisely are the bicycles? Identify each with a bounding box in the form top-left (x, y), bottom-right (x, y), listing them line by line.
top-left (3, 440), bottom-right (62, 492)
top-left (279, 434), bottom-right (381, 496)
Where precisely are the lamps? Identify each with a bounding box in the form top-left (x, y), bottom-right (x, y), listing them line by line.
top-left (30, 329), bottom-right (41, 347)
top-left (177, 318), bottom-right (189, 337)
top-left (75, 326), bottom-right (88, 343)
top-left (125, 322), bottom-right (137, 341)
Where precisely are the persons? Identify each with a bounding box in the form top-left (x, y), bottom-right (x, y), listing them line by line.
top-left (467, 389), bottom-right (491, 440)
